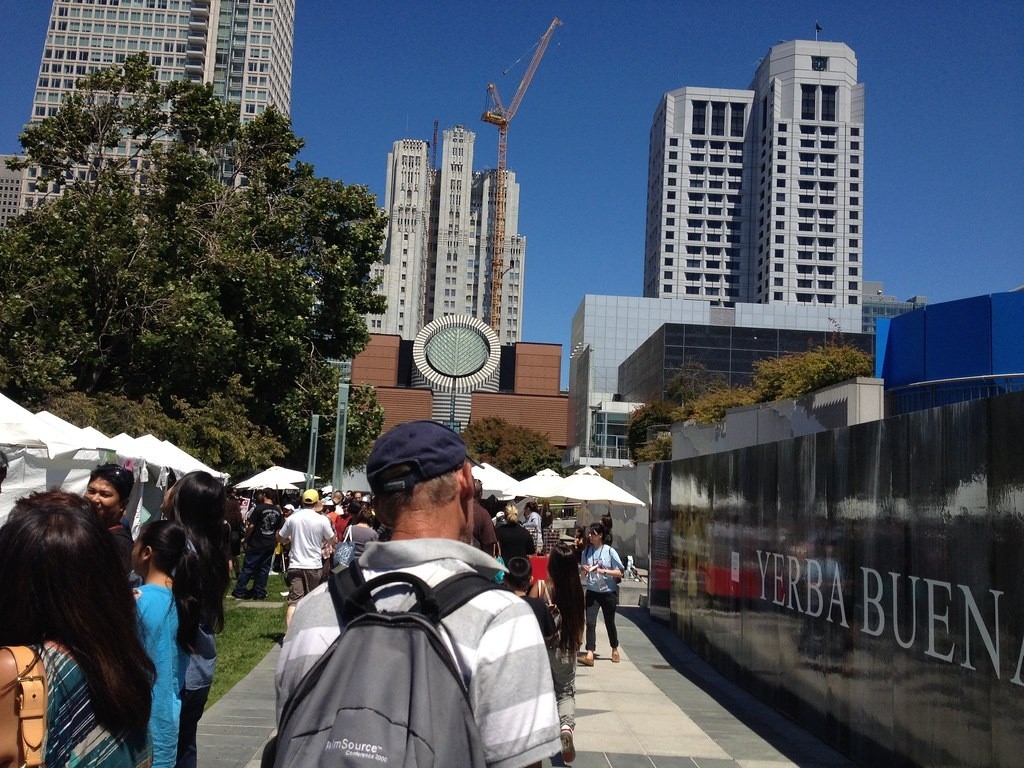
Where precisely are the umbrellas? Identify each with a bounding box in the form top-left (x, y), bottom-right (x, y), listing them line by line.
top-left (234, 459), bottom-right (646, 576)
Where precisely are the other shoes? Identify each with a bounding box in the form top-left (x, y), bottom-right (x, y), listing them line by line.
top-left (268, 570), bottom-right (278, 575)
top-left (231, 592), bottom-right (252, 601)
top-left (612, 652), bottom-right (620, 662)
top-left (577, 654), bottom-right (594, 666)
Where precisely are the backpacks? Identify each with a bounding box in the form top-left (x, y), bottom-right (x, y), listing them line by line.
top-left (270, 559), bottom-right (517, 768)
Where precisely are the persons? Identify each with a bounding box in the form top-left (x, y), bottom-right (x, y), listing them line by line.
top-left (0, 448), bottom-right (627, 768)
top-left (263, 418), bottom-right (570, 768)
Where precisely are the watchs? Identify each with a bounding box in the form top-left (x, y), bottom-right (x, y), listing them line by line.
top-left (603, 569), bottom-right (607, 574)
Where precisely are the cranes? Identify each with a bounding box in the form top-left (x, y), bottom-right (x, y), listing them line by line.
top-left (480, 17), bottom-right (567, 340)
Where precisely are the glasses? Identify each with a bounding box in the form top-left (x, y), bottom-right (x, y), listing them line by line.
top-left (98, 467), bottom-right (129, 488)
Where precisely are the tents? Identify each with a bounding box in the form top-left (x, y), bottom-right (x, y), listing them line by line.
top-left (1, 392), bottom-right (232, 540)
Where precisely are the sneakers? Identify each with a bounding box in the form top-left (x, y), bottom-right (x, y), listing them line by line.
top-left (560, 725), bottom-right (576, 762)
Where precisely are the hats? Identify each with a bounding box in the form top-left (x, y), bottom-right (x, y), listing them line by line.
top-left (359, 496), bottom-right (371, 504)
top-left (366, 420), bottom-right (485, 496)
top-left (495, 511), bottom-right (505, 519)
top-left (283, 504), bottom-right (294, 512)
top-left (321, 497), bottom-right (334, 506)
top-left (302, 489), bottom-right (319, 505)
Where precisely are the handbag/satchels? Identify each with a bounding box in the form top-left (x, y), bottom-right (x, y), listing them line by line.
top-left (331, 525), bottom-right (355, 574)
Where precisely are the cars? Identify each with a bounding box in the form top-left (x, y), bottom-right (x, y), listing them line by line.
top-left (648, 553), bottom-right (788, 614)
